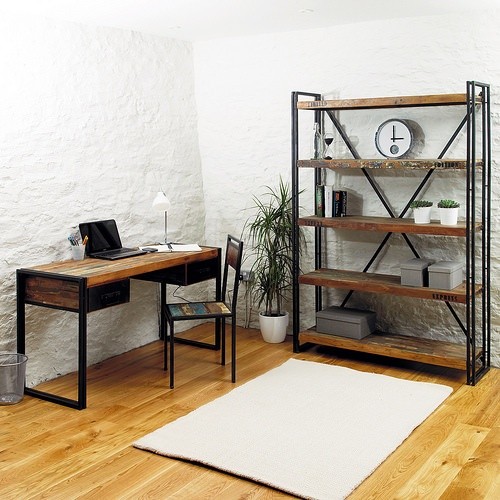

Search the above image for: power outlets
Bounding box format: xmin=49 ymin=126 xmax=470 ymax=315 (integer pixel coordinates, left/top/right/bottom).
xmin=240 ymin=271 xmax=254 ymax=281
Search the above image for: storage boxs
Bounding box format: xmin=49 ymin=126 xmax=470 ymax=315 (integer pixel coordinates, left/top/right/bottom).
xmin=315 ymin=184 xmax=348 ymax=217
xmin=316 ymin=306 xmax=377 ymax=340
xmin=400 ymin=258 xmax=464 ymax=291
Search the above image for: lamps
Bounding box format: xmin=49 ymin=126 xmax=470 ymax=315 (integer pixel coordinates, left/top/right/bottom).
xmin=152 ymin=186 xmax=171 ymax=245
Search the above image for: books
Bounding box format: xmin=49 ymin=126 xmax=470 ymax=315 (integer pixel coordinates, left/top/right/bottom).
xmin=316 ymin=184 xmax=347 ymax=218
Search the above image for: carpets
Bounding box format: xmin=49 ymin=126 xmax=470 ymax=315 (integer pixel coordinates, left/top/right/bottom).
xmin=129 ymin=358 xmax=453 ymax=500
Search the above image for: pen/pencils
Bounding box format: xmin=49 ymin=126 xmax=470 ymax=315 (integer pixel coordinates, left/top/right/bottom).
xmin=67 ymin=236 xmax=89 ymax=247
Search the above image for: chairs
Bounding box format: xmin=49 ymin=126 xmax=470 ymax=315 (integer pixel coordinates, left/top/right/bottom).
xmin=164 ymin=234 xmax=244 ymax=389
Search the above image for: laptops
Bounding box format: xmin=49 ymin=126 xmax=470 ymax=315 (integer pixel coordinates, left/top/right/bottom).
xmin=79 ymin=219 xmax=146 ymax=260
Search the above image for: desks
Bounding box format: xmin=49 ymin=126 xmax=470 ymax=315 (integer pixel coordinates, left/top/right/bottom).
xmin=16 ymin=243 xmax=222 ymax=411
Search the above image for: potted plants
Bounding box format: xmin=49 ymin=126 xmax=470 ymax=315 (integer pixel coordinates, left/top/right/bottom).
xmin=410 ymin=201 xmax=433 ymax=224
xmin=239 ymin=173 xmax=313 ymax=344
xmin=437 ymin=199 xmax=460 ymax=225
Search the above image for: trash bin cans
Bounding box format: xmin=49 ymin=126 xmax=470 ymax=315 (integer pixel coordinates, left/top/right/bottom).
xmin=0 ymin=353 xmax=28 ymax=406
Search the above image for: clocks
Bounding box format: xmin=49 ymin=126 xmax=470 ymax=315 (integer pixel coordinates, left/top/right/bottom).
xmin=374 ymin=118 xmax=413 ymax=158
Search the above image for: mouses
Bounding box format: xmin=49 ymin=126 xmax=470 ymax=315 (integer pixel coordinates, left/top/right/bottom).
xmin=142 ymin=247 xmax=158 ymax=253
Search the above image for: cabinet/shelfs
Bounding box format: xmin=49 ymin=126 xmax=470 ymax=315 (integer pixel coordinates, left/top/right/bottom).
xmin=291 ymin=81 xmax=490 ymax=387
